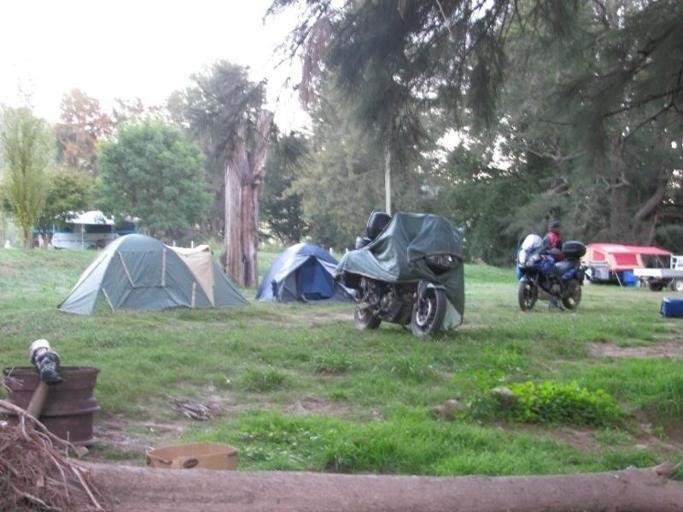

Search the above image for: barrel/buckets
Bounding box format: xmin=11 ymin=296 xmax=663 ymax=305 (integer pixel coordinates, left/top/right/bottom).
xmin=5 ymin=367 xmax=100 ymax=445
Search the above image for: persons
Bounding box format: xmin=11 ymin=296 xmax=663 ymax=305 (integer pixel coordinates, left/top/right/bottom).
xmin=543 ymin=220 xmax=565 ymax=311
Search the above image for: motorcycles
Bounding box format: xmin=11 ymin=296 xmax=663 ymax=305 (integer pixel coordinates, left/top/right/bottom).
xmin=340 ymin=208 xmax=465 ymax=341
xmin=513 ymin=230 xmax=587 ymax=311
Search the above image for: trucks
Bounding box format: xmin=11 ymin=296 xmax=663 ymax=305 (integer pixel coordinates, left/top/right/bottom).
xmin=630 ymin=252 xmax=682 ymax=292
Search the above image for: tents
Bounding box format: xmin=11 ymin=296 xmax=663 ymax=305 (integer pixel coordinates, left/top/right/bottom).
xmin=57 ymin=234 xmax=214 ymax=317
xmin=255 ymin=243 xmax=357 ymax=301
xmin=165 ymin=244 xmax=249 ymax=306
